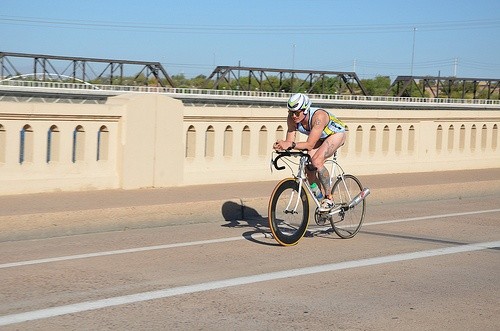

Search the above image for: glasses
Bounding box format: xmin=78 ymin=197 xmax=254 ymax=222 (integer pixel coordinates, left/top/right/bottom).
xmin=288 ymin=109 xmax=303 ymax=117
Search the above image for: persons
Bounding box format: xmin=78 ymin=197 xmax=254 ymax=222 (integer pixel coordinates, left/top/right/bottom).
xmin=273 ymin=93 xmax=349 ymax=212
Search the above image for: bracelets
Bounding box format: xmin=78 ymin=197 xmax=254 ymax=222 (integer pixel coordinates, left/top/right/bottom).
xmin=292 ymin=142 xmax=295 ymax=148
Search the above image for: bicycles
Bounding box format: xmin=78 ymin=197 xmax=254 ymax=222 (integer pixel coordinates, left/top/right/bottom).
xmin=268 ymin=141 xmax=371 ymax=247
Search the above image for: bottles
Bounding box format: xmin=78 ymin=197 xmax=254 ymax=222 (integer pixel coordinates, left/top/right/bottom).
xmin=310 ymin=182 xmax=323 ymax=201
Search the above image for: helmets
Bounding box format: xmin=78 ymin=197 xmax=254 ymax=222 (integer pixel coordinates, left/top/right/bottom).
xmin=287 ymin=92 xmax=311 ymax=115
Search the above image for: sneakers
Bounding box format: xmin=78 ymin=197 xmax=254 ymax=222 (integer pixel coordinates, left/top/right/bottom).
xmin=318 ymin=211 xmax=326 ymax=225
xmin=318 ymin=199 xmax=335 ymax=211
